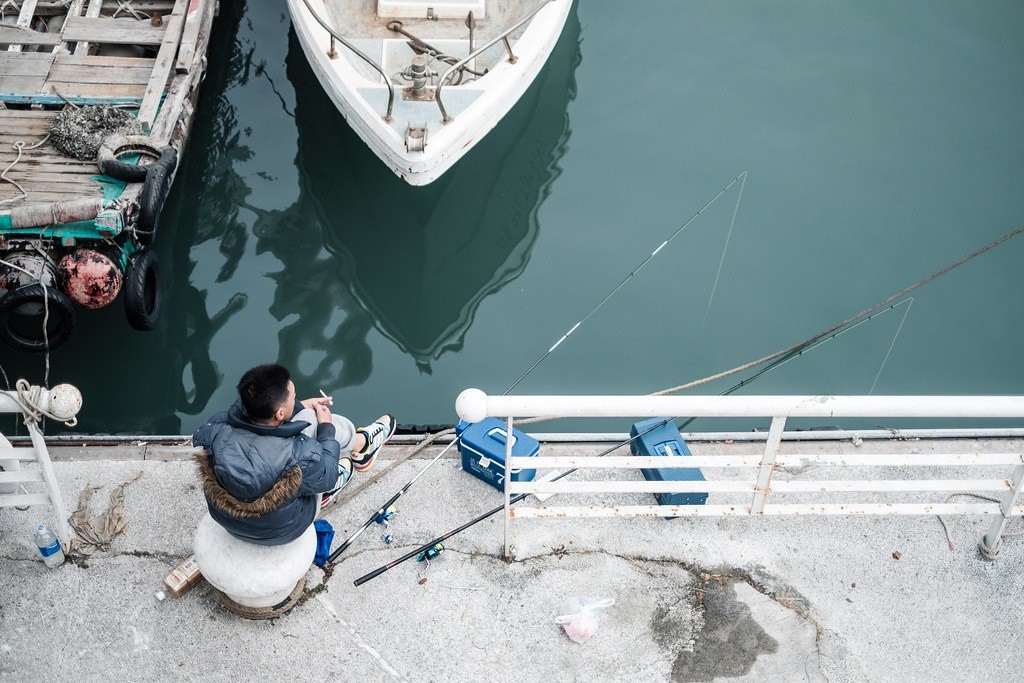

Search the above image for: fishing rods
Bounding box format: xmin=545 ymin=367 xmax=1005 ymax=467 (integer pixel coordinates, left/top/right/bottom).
xmin=326 ymin=165 xmax=749 ymax=566
xmin=352 ymin=221 xmax=1024 ymax=588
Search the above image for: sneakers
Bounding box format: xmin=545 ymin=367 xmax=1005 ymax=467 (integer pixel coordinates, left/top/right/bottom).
xmin=351 ymin=413 xmax=396 ymax=472
xmin=319 ymin=456 xmax=355 ymax=508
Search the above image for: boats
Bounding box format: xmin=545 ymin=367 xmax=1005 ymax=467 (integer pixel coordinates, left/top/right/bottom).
xmin=286 ymin=0 xmax=577 ymax=189
xmin=285 ymin=26 xmax=585 ymax=377
xmin=0 ymin=0 xmax=226 ymax=359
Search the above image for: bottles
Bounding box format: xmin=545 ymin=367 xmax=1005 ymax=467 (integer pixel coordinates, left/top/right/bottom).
xmin=33 ymin=525 xmax=65 ymax=569
xmin=155 ymin=555 xmax=203 ymax=601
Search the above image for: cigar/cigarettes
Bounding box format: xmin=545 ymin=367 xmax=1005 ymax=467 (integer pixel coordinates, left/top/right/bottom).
xmin=319 ymin=389 xmax=333 ymax=408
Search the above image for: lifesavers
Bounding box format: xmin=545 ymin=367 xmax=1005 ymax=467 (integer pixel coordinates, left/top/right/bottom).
xmin=136 ymin=164 xmax=168 ymax=245
xmin=123 ymin=250 xmax=163 ymax=331
xmin=0 ymin=284 xmax=77 ymax=352
xmin=97 ymin=133 xmax=179 ymax=183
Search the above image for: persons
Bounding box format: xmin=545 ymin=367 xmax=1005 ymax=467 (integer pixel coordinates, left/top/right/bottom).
xmin=192 ymin=365 xmax=397 ymax=545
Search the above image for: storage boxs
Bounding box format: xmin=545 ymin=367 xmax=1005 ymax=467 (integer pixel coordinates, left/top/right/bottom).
xmin=458 ymin=419 xmax=540 ymax=496
xmin=630 ymin=417 xmax=708 ymax=520
